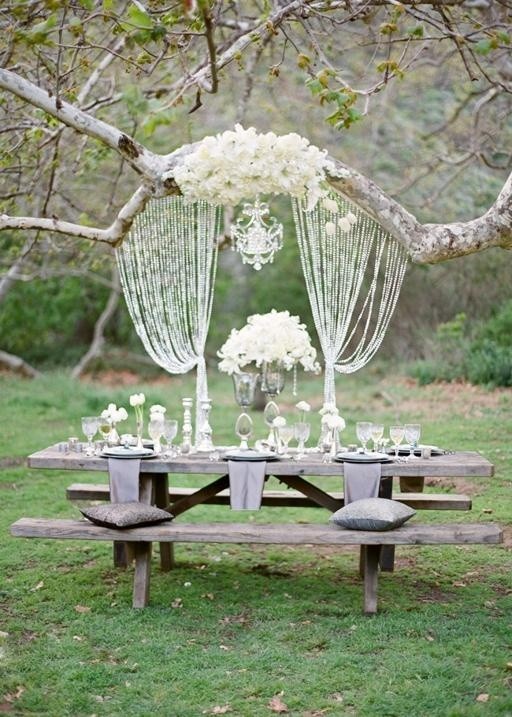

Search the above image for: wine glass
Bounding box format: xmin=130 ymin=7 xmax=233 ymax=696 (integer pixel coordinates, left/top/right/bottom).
xmin=390 ymin=422 xmax=421 ymax=461
xmin=260 ymin=359 xmax=286 ymax=443
xmin=274 ymin=421 xmax=312 ymax=459
xmin=161 ymin=419 xmax=179 ymax=453
xmin=354 ymin=419 xmax=384 ymax=452
xmin=80 ymin=413 xmax=113 ymax=454
xmin=230 ymin=372 xmax=257 ymax=450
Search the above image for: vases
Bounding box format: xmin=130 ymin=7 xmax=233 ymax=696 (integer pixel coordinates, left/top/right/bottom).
xmin=329 ymin=431 xmax=340 ymax=457
xmin=252 ymin=361 xmax=287 ymax=403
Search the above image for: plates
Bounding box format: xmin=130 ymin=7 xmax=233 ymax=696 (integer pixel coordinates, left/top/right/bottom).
xmin=223 ymin=450 xmax=275 ymax=462
xmin=334 ymin=450 xmax=390 ymax=463
xmin=98 ymin=445 xmax=160 ymax=459
xmin=119 ymin=436 xmax=154 ymax=447
xmin=392 ymin=443 xmax=441 ymax=455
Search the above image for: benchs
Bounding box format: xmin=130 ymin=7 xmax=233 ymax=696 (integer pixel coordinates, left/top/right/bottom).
xmin=9 ymin=514 xmax=506 ymax=615
xmin=65 ymin=479 xmax=474 ymax=574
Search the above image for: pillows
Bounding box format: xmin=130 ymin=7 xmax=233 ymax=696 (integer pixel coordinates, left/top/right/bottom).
xmin=327 ymin=495 xmax=419 ymax=532
xmin=77 ymin=501 xmax=177 ymax=530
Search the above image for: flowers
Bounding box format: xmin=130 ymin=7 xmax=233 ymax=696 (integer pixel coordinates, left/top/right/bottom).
xmin=318 ymin=399 xmax=339 ymax=418
xmin=100 ymin=402 xmax=130 ymax=427
xmin=216 ymin=304 xmax=322 ymax=376
xmin=146 ymin=405 xmax=166 ymax=425
xmin=324 ymin=415 xmax=345 ymax=437
xmin=130 ymin=390 xmax=147 ymax=427
xmin=271 ymin=415 xmax=287 ymax=433
xmin=294 ymin=397 xmax=310 ymax=425
xmin=171 ymin=120 xmax=359 ymax=237
